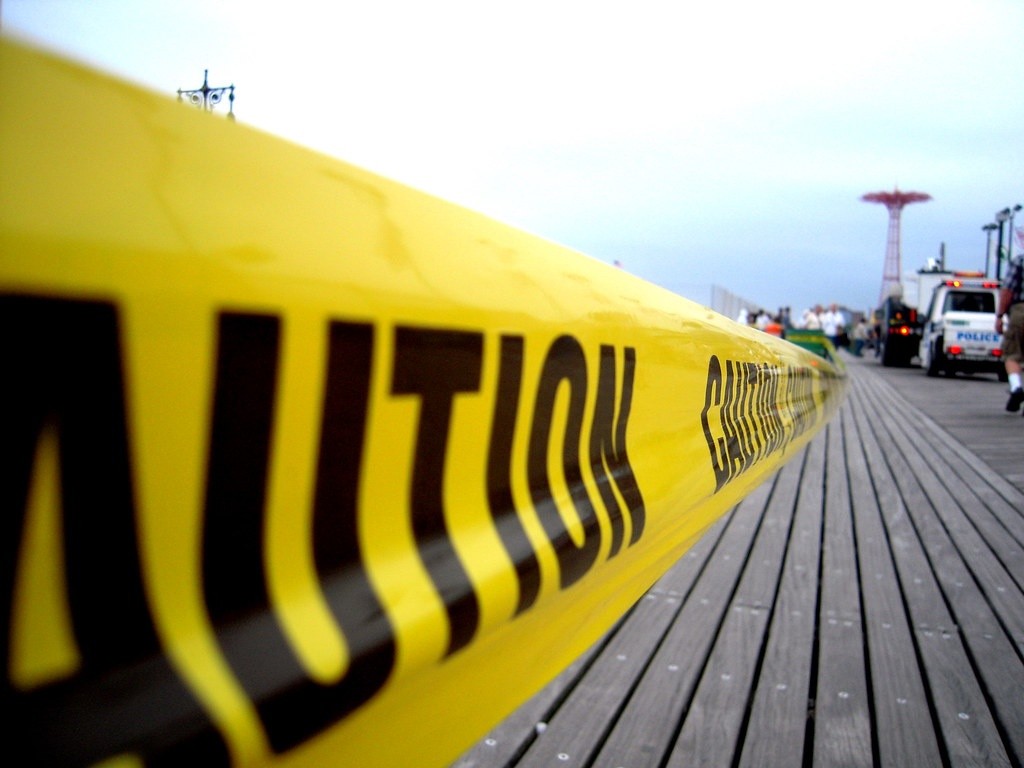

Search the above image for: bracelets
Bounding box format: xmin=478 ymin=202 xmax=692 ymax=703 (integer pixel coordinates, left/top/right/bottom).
xmin=996 ymin=313 xmax=1003 ymax=318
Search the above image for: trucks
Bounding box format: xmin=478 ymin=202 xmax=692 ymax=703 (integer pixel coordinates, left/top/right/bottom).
xmin=874 ymin=266 xmax=957 ymax=368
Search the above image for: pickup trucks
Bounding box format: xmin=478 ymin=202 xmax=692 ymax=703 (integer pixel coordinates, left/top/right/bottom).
xmin=919 ymin=280 xmax=1012 ymax=384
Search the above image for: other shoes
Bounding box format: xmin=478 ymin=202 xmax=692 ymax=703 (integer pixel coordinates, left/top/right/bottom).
xmin=1006 ymin=388 xmax=1024 ymax=411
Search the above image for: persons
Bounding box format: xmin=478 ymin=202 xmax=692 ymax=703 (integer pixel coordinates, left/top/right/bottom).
xmin=746 ymin=303 xmax=882 ymax=358
xmin=995 ymin=253 xmax=1024 ymax=417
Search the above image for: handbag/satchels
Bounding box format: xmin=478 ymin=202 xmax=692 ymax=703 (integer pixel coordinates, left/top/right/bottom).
xmin=834 ymin=333 xmax=851 ymax=348
